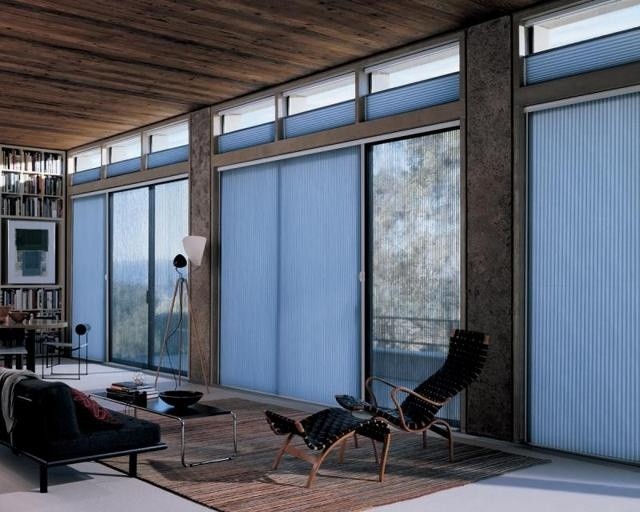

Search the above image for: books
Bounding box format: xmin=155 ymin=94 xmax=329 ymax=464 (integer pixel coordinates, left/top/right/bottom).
xmin=107 ymin=381 xmax=160 ymax=401
xmin=0 ymin=288 xmax=61 ymax=354
xmin=0 ymin=149 xmax=63 ymax=218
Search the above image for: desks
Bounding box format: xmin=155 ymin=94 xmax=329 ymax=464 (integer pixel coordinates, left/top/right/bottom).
xmin=0 ymin=322 xmax=69 ymax=373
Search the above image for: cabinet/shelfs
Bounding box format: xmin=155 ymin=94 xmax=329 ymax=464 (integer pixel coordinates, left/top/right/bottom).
xmin=0 ymin=144 xmax=66 ymax=357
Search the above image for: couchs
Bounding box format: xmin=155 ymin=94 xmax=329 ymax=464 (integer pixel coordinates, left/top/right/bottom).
xmin=0 ymin=365 xmax=169 ymax=493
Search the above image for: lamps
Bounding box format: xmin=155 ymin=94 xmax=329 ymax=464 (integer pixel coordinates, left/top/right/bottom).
xmin=155 ymin=235 xmax=210 ymax=394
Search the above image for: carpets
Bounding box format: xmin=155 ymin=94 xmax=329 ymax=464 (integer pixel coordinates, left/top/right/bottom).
xmin=82 ymin=382 xmax=551 ymax=512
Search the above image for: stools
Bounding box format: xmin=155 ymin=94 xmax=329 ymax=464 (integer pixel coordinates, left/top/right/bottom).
xmin=0 ymin=346 xmax=29 ymax=370
xmin=263 ymin=407 xmax=391 ymax=487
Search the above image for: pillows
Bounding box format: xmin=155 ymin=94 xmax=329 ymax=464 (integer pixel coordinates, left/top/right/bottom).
xmin=72 ymin=387 xmax=112 ymax=420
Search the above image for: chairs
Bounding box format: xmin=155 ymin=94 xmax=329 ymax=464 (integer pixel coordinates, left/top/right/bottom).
xmin=335 ymin=328 xmax=490 ymax=458
xmin=43 ymin=324 xmax=91 ymax=380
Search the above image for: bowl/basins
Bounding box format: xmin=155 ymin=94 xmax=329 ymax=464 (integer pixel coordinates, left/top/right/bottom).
xmin=158 ymin=391 xmax=202 ymax=407
xmin=9 ymin=311 xmax=27 ymax=322
